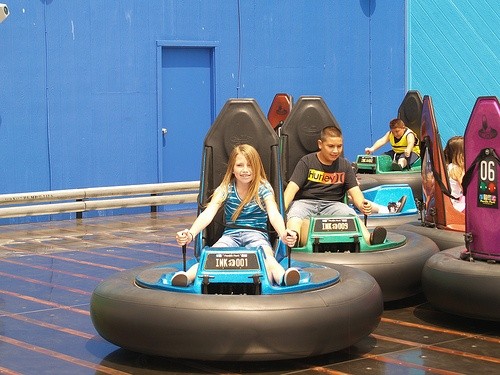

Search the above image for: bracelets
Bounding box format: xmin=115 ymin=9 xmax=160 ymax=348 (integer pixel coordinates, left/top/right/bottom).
xmin=190 ymin=232 xmax=193 ymax=243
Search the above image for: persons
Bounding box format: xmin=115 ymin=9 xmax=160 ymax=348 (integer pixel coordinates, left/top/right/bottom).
xmin=349 ymin=176 xmax=406 ymax=215
xmin=282 ymin=126 xmax=387 ymax=248
xmin=442 ymin=136 xmax=466 ymax=215
xmin=171 ymin=144 xmax=301 ymax=288
xmin=364 ymin=119 xmax=420 ymax=171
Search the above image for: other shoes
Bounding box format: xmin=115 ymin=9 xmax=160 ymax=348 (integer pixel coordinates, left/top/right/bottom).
xmin=391 ymin=162 xmax=404 ymax=171
xmin=387 ymin=195 xmax=408 ymax=214
xmin=280 ymin=267 xmax=301 ymax=286
xmin=369 ymin=225 xmax=387 ymax=245
xmin=171 ymin=270 xmax=189 ymax=287
xmin=352 ymin=162 xmax=357 ymax=171
xmin=286 ymin=231 xmax=299 ymax=249
